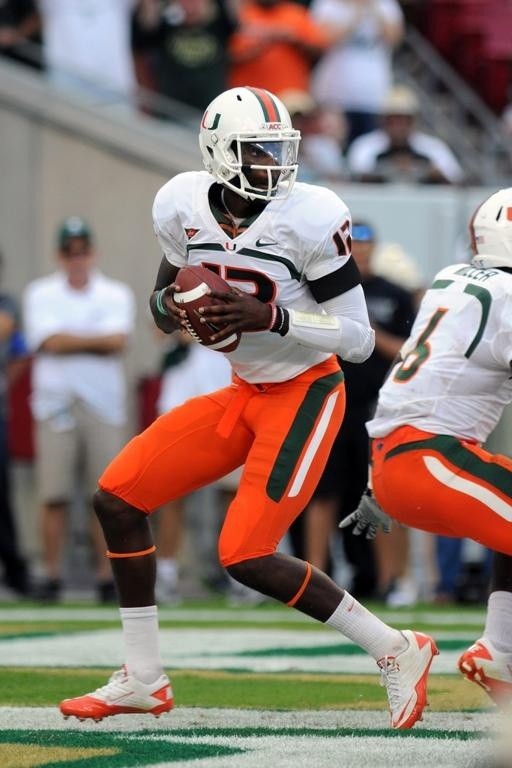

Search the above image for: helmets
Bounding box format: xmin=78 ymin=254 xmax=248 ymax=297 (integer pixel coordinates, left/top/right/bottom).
xmin=198 ymin=87 xmax=301 ymax=202
xmin=469 ymin=184 xmax=512 ymax=272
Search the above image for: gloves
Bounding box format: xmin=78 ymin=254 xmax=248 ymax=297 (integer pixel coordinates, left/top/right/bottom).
xmin=337 ymin=489 xmax=408 ymax=542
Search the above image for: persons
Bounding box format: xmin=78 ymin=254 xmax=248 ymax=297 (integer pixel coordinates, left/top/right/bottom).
xmin=340 ymin=189 xmax=512 ymax=714
xmin=60 ymin=86 xmax=440 ymax=731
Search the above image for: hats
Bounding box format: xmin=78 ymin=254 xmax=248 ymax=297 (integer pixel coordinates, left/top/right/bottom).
xmin=55 ymin=216 xmax=89 ymax=240
xmin=378 ymin=87 xmax=419 ymax=119
xmin=348 ymin=222 xmax=376 ymax=242
xmin=277 ymin=84 xmax=318 ymax=122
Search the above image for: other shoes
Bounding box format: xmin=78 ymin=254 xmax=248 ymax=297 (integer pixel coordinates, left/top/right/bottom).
xmin=153 ymin=559 xmax=182 ymax=604
xmin=38 ymin=579 xmax=66 ymax=606
xmin=228 ymin=580 xmax=270 ymax=609
xmin=455 ymin=561 xmax=488 ymax=605
xmin=98 ymin=580 xmax=119 ymax=605
xmin=386 ymin=568 xmax=443 ymax=608
xmin=349 ymin=563 xmax=380 ymax=597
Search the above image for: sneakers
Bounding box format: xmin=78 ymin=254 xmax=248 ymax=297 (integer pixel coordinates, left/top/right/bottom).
xmin=458 ymin=636 xmax=512 ymax=727
xmin=57 ymin=662 xmax=174 ymax=721
xmin=378 ymin=625 xmax=444 ymax=732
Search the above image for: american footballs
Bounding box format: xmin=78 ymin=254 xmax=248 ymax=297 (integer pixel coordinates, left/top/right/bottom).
xmin=171 ymin=264 xmax=240 ymax=352
